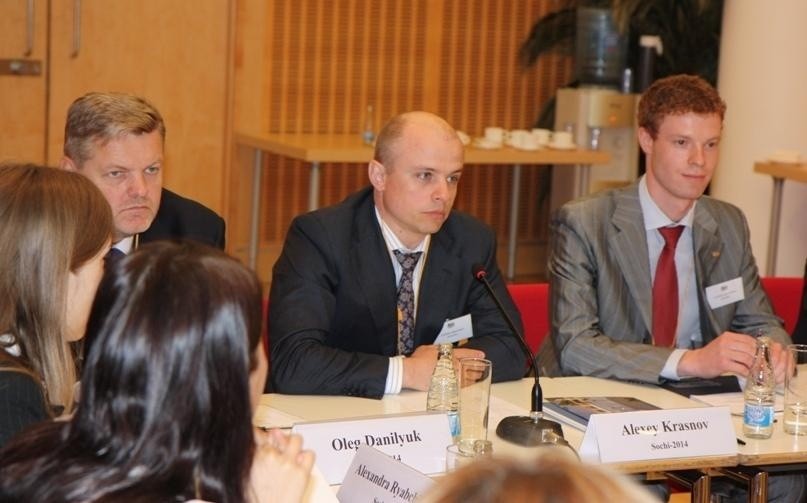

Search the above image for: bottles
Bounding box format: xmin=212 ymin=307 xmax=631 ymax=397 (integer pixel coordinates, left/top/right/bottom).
xmin=427 ymin=343 xmax=458 ymax=412
xmin=362 ymin=105 xmax=374 ymax=145
xmin=742 ymin=336 xmax=774 ymax=439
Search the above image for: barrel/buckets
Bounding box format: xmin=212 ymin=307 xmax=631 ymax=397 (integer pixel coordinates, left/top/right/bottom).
xmin=576 ymin=6 xmax=630 ymax=85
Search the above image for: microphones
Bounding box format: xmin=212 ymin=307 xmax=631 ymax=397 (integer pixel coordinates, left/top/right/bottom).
xmin=470 ymin=261 xmax=565 ymax=448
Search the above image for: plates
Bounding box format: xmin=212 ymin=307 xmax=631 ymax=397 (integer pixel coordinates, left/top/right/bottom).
xmin=478 ymin=141 xmax=578 ymax=151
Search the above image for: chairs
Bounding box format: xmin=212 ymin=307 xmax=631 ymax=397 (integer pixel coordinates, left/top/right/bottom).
xmin=506 ymin=283 xmax=550 ymax=354
xmin=761 ymin=278 xmax=804 ymax=336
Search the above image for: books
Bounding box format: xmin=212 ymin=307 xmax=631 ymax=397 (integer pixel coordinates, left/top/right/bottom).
xmin=541 ymin=397 xmax=663 ymax=432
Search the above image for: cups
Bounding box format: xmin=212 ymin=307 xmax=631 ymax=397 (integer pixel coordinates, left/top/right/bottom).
xmin=446 ymin=444 xmax=482 ymax=475
xmin=458 ymin=357 xmax=492 ymax=453
xmin=784 ymin=344 xmax=807 ymax=436
xmin=484 ymin=127 xmax=573 ymax=146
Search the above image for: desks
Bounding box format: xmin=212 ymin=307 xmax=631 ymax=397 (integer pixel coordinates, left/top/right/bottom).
xmin=753 ymin=161 xmax=807 ymax=278
xmin=252 ymin=364 xmax=807 ymax=503
xmin=237 ymin=130 xmax=613 ymax=280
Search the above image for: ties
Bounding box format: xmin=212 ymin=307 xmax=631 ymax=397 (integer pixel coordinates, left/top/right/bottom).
xmin=390 ymin=249 xmax=424 ymax=359
xmin=650 ymin=225 xmax=686 ymax=351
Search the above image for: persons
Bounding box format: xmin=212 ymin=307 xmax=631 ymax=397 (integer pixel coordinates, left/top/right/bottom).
xmin=0 ymin=242 xmax=317 ymax=503
xmin=63 ymin=89 xmax=225 ymax=378
xmin=411 ymin=445 xmax=659 ymax=502
xmin=261 ymin=110 xmax=527 ymax=399
xmin=524 ymin=74 xmax=805 ymax=503
xmin=0 ymin=163 xmax=113 ymax=441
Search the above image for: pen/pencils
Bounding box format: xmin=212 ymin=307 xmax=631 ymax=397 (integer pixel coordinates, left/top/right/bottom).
xmin=737 ymin=438 xmax=746 ymax=445
